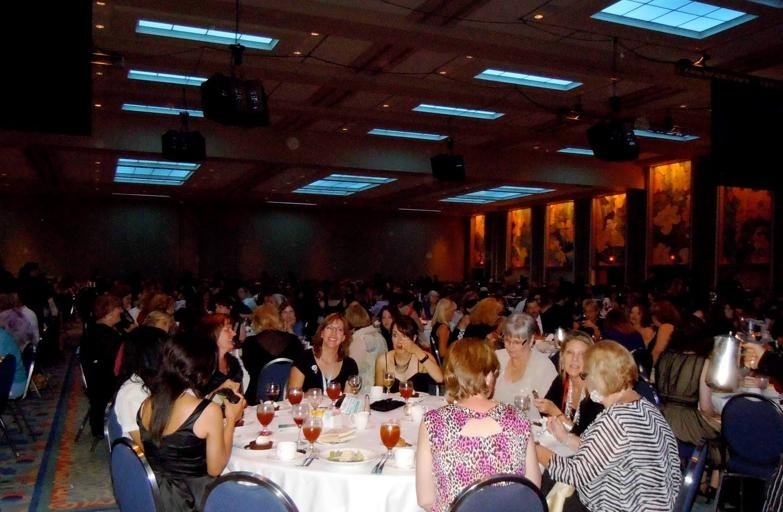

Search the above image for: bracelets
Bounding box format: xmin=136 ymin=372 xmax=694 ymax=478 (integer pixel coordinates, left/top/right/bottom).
xmin=419 ymin=352 xmax=430 ymax=363
xmin=557 ymin=412 xmax=564 ymax=420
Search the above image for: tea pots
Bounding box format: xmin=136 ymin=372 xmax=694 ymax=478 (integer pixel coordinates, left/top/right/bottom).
xmin=706 ymin=331 xmax=750 ymax=392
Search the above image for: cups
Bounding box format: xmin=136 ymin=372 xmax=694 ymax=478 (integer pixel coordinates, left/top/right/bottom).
xmin=350 ymin=412 xmax=369 ymax=431
xmin=395 ymin=449 xmax=415 ymax=468
xmin=411 ymin=405 xmax=428 ymax=423
xmin=276 ymin=441 xmax=297 ymax=461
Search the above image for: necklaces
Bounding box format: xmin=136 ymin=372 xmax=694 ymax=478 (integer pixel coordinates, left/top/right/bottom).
xmin=392 ymin=350 xmax=416 ymax=375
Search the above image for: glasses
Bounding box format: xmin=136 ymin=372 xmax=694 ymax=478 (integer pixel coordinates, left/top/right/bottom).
xmin=579 ymin=371 xmax=590 ymax=380
xmin=499 ymin=335 xmax=528 ymax=347
xmin=325 ymin=327 xmax=344 ymax=335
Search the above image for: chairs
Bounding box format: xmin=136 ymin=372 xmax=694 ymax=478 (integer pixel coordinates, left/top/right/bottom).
xmin=258 ymin=357 xmax=294 ymax=403
xmin=21 ymin=342 xmax=38 ymax=441
xmin=200 ymin=472 xmax=298 ymax=512
xmin=73 ymin=346 xmax=92 ymax=443
xmin=713 ymin=395 xmax=782 ymax=511
xmin=108 ymin=437 xmax=161 ymax=512
xmin=0 ymin=354 xmax=19 ymax=459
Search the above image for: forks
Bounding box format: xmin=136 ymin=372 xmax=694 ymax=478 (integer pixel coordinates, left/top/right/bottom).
xmin=305 ymin=454 xmax=317 ymax=466
xmin=377 ymin=457 xmax=389 ymax=474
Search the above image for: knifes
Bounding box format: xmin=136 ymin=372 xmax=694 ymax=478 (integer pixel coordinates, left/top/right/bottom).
xmin=302 ymin=454 xmax=311 ymax=466
xmin=372 ymin=455 xmax=385 ymax=472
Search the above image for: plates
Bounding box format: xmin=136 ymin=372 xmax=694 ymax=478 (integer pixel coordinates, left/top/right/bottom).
xmin=321 ymin=448 xmax=377 ymax=463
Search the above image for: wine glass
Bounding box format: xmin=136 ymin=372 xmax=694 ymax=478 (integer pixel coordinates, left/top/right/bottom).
xmin=514 ymin=395 xmax=528 ymax=412
xmin=399 ymin=382 xmax=413 ymax=406
xmin=292 ymin=404 xmax=309 ymax=445
xmin=327 ymin=382 xmax=340 ymax=407
xmin=266 ymin=383 xmax=280 ymax=405
xmin=287 ymin=387 xmax=302 ymax=405
xmin=348 ymin=375 xmax=362 ymax=394
xmin=756 ymin=378 xmax=768 ymax=393
xmin=380 ymin=423 xmax=400 ymax=456
xmin=383 ymin=372 xmax=395 ymax=395
xmin=256 ymin=404 xmax=274 ymax=436
xmin=308 ymin=388 xmax=322 ymax=410
xmin=302 ymin=417 xmax=322 ymax=450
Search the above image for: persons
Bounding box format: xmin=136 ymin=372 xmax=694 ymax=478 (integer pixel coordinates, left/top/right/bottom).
xmin=17 ymin=261 xmax=58 ymax=335
xmin=513 ymin=290 xmax=541 ymax=314
xmin=238 ymin=285 xmax=257 ymax=310
xmin=346 ymin=304 xmax=389 ymax=384
xmin=203 ymin=281 xmax=222 ymax=316
xmin=748 ymin=296 xmax=768 ymax=318
xmin=422 ymin=289 xmax=441 ymax=318
xmin=630 ymin=301 xmax=655 ymax=348
xmin=367 ymin=290 xmax=389 ymax=315
xmin=258 ymin=291 xmax=288 ymax=307
xmin=285 ymin=313 xmax=358 ymax=401
xmin=768 ymin=312 xmax=783 ymax=357
xmin=111 ymin=281 xmax=138 ymax=334
xmin=533 ymin=329 xmax=605 ymax=437
xmin=338 ymin=283 xmax=356 ymax=309
xmin=140 ymin=284 xmax=159 ymax=320
xmin=1 ymin=291 xmax=35 ymax=357
xmin=741 ymin=340 xmax=783 ymax=394
xmin=114 ymin=312 xmax=175 ymax=380
xmin=282 ymin=302 xmax=304 ymax=340
xmin=106 ymin=326 xmax=170 ymax=493
xmin=518 ymin=271 xmax=531 ymax=291
xmin=13 ymin=290 xmax=44 ymax=346
xmin=665 ymin=274 xmax=691 ymax=306
xmin=485 ymin=313 xmax=561 ymax=411
xmin=574 ymin=298 xmax=606 ymax=334
xmin=531 ymin=339 xmax=686 ymax=511
xmin=465 ymin=285 xmax=504 ymax=346
xmin=597 ymin=310 xmax=651 ymax=375
xmin=429 ymin=298 xmax=458 ymax=365
xmin=521 ymin=299 xmax=556 ymax=336
xmin=77 ymin=293 xmax=124 ymax=439
xmin=649 ymin=302 xmax=740 ymax=384
xmin=379 ymin=303 xmax=404 ymax=350
xmin=136 ymin=339 xmax=248 ymax=511
xmin=150 ymin=294 xmax=180 ymax=343
xmin=191 ymin=312 xmax=251 ymax=396
xmin=242 ymin=303 xmax=306 ymax=406
xmin=413 ymin=337 xmax=542 ymax=510
xmin=374 ymin=316 xmax=444 ymax=393
xmin=397 ymin=291 xmax=424 ymax=332
xmin=647 ymin=290 xmax=683 ymax=327
xmin=1 ymin=327 xmax=29 ymax=405
xmin=212 ymin=299 xmax=248 ymax=346
